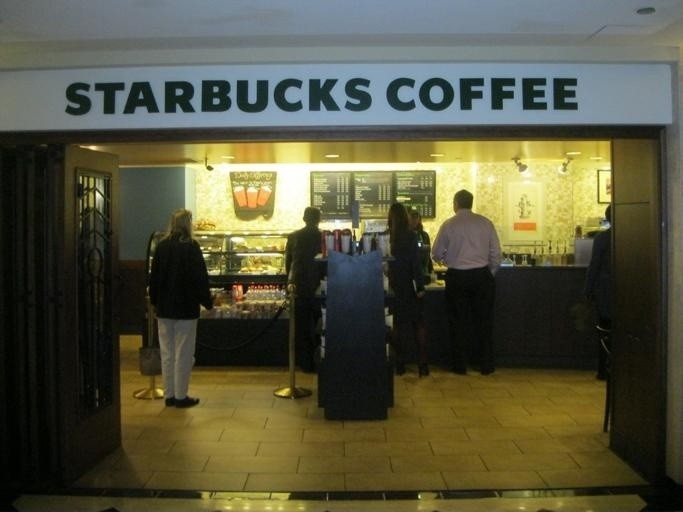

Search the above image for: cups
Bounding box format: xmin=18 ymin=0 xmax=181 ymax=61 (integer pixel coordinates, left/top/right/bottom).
xmin=234 ymin=186 xmax=272 ymax=209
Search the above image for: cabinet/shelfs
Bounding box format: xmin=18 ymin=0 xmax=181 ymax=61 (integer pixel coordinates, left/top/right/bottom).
xmin=147 ymin=231 xmax=292 ymax=366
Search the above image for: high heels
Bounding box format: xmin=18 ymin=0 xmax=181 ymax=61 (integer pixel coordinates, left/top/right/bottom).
xmin=397 ymin=361 xmax=429 ymax=378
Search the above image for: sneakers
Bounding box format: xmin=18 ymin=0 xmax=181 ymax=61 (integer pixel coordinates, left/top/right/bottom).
xmin=165 ymin=396 xmax=199 ymax=408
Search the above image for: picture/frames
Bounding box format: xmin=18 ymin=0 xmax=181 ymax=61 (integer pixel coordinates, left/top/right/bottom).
xmin=598 ymin=169 xmax=611 ymax=203
xmin=501 ymin=176 xmax=546 ymax=245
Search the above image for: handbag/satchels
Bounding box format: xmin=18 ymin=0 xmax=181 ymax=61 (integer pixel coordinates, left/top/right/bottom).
xmin=138 ymin=347 xmax=163 ymax=376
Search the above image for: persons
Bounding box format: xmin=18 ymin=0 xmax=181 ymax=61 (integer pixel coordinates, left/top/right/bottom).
xmin=150 ymin=209 xmax=215 ymax=409
xmin=284 ymin=207 xmax=326 ymax=375
xmin=380 ymin=204 xmax=430 ymax=378
xmin=433 ymin=188 xmax=501 ymax=375
xmin=583 ymin=204 xmax=614 ymax=381
xmin=408 ymin=209 xmax=433 ymax=286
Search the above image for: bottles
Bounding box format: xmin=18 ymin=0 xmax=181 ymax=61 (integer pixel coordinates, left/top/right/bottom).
xmin=232 ymin=282 xmax=286 ymax=319
xmin=531 ymin=240 xmax=568 ymax=266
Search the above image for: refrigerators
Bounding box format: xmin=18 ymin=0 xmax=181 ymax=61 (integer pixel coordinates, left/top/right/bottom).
xmin=145 ymin=229 xmax=295 ymax=276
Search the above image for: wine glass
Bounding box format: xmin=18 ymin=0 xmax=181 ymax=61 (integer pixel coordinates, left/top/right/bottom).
xmin=502 ymin=245 xmax=512 ymax=263
